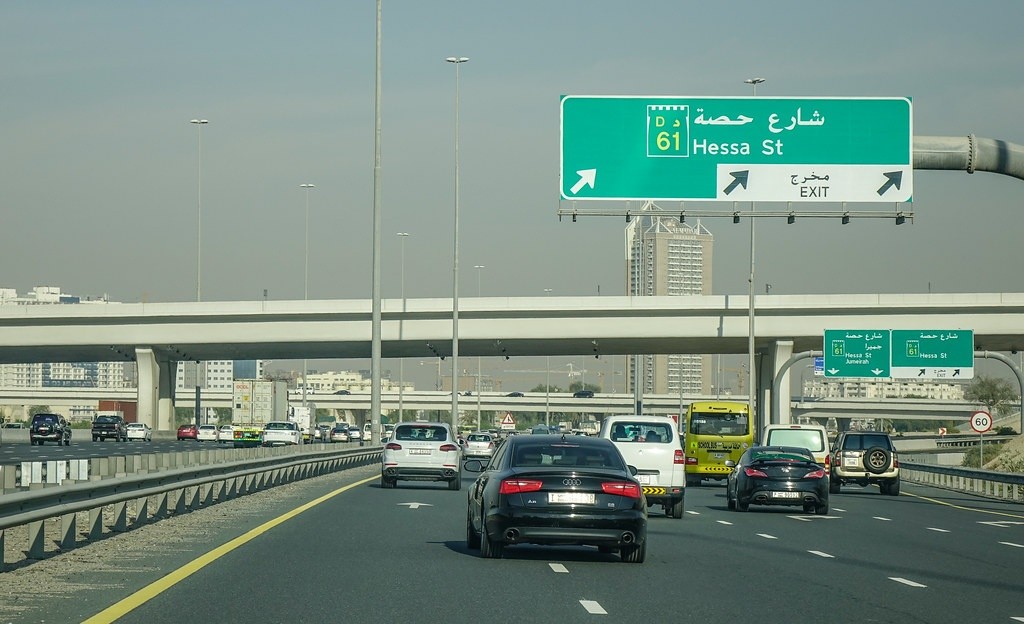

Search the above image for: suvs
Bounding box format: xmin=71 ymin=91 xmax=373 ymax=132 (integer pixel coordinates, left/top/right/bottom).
xmin=831 ymin=432 xmax=900 ymax=496
xmin=31 ymin=414 xmax=73 ymax=447
xmin=574 ymin=391 xmax=595 ymax=398
xmin=263 ymin=421 xmax=305 ymax=447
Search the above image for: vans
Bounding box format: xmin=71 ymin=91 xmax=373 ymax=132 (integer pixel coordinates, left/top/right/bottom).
xmin=363 ymin=424 xmax=386 ymax=440
xmin=762 ymin=425 xmax=831 ymax=493
xmin=383 ymin=424 xmax=394 ymax=438
xmin=600 ymin=415 xmax=685 ymax=518
xmin=295 ymin=387 xmax=316 ymax=396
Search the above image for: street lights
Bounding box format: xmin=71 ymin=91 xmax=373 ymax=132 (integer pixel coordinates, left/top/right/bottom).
xmin=474 ymin=266 xmax=487 ymax=433
xmin=746 ymin=77 xmax=767 ymax=402
xmin=397 ymin=232 xmax=409 ymax=423
xmin=446 ymin=56 xmax=469 ymax=441
xmin=192 ymin=120 xmax=208 ymax=302
xmin=300 ymin=182 xmax=316 ymax=299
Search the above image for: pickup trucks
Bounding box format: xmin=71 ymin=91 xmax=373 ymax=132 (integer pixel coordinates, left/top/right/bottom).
xmin=92 ymin=415 xmax=128 ymax=442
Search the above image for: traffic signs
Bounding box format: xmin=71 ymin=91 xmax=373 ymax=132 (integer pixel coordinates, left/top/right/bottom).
xmin=824 ymin=329 xmax=890 ymax=379
xmin=891 ymin=330 xmax=976 ymax=381
xmin=560 ymin=93 xmax=913 ymax=203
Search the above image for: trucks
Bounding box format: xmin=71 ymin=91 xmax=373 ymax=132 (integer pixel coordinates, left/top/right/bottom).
xmin=233 ymin=379 xmax=291 ymax=449
xmin=290 ymin=407 xmax=316 ymax=444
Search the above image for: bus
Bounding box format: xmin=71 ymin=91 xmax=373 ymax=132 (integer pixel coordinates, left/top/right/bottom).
xmin=684 ymin=402 xmax=754 ymax=485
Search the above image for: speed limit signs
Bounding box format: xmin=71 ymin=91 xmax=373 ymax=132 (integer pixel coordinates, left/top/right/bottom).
xmin=971 ymin=411 xmax=993 ymax=432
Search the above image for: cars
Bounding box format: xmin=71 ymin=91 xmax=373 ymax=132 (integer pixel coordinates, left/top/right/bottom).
xmin=467 ymin=435 xmax=648 ymax=564
xmin=349 ymin=428 xmax=363 ymax=441
xmin=4 ymin=423 xmax=26 ymax=430
xmin=219 ymin=425 xmax=234 ymax=444
xmin=507 ymin=392 xmax=525 ymax=397
xmin=726 ymin=446 xmax=830 ymax=514
xmin=531 ymin=422 xmax=598 ymax=438
xmin=178 ymin=425 xmax=200 ymax=441
xmin=331 ymin=429 xmax=353 ymax=443
xmin=198 ymin=425 xmax=220 ymax=442
xmin=127 ymin=423 xmax=153 ymax=442
xmin=463 ymin=433 xmax=495 ymax=460
xmin=381 ymin=423 xmax=462 ymax=491
xmin=315 ymin=427 xmax=327 ymax=442
xmin=334 ymin=390 xmax=351 ymax=396
